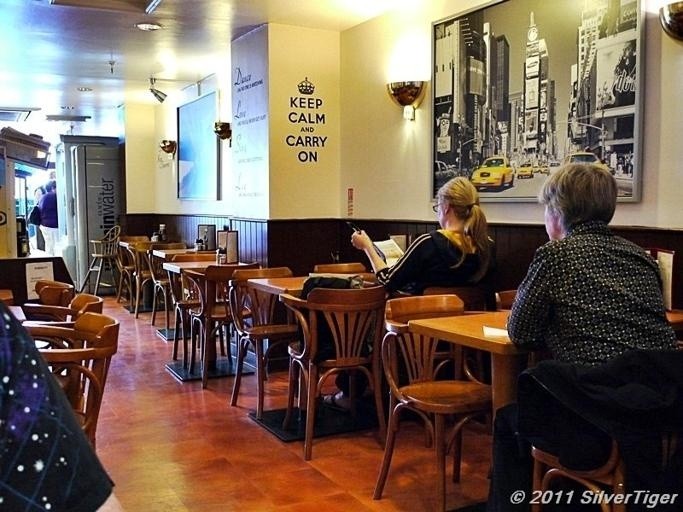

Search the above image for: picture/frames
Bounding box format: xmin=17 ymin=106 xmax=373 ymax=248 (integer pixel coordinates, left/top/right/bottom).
xmin=428 ymin=1 xmax=644 ymax=203
xmin=177 ymin=88 xmax=221 ymax=200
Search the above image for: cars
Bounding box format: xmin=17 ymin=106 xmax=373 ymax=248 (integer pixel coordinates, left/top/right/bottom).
xmin=563 ymin=153 xmax=614 ymax=175
xmin=548 ymin=162 xmax=560 ymax=175
xmin=518 ymin=164 xmax=533 ymax=179
xmin=471 ymin=156 xmax=514 ymax=191
xmin=533 ymin=165 xmax=540 ymax=172
xmin=540 ymin=165 xmax=549 ymax=174
xmin=433 ymin=160 xmax=456 ymax=182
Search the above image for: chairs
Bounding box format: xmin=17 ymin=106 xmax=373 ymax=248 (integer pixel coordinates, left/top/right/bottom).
xmin=79 ymin=225 xmax=122 ymax=299
xmin=1 ymin=276 xmax=114 ymax=455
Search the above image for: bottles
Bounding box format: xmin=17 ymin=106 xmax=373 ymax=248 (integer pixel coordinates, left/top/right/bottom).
xmin=194 ymin=239 xmax=202 ymax=251
xmin=158 ymin=224 xmax=168 ymax=241
xmin=216 ymin=249 xmax=226 ymax=264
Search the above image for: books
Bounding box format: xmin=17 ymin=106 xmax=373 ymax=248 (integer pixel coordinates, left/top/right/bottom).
xmin=347 ymin=221 xmax=404 ymax=271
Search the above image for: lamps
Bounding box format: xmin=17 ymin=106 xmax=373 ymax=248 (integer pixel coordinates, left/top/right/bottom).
xmin=149 ymin=78 xmax=167 ymax=104
xmin=159 ymin=138 xmax=176 ymax=159
xmin=386 ymin=80 xmax=427 ymax=121
xmin=212 ymin=121 xmax=231 ymax=148
xmin=658 ymin=1 xmax=683 ymax=44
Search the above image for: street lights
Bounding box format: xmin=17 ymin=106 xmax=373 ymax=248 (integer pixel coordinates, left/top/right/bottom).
xmin=456 ymin=111 xmax=465 ymax=177
xmin=596 ymin=81 xmax=608 ymax=162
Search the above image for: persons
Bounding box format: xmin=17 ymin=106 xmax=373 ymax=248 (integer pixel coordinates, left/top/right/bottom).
xmin=317 ymin=175 xmax=498 ymax=413
xmin=25 ymin=185 xmax=45 ymax=251
xmin=487 ymin=159 xmax=675 ymax=511
xmin=0 ymin=301 xmax=123 ymax=511
xmin=38 ymin=180 xmax=58 ymax=254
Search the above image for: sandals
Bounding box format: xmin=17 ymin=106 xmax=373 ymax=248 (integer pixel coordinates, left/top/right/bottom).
xmin=321 ymin=392 xmax=350 ymax=416
